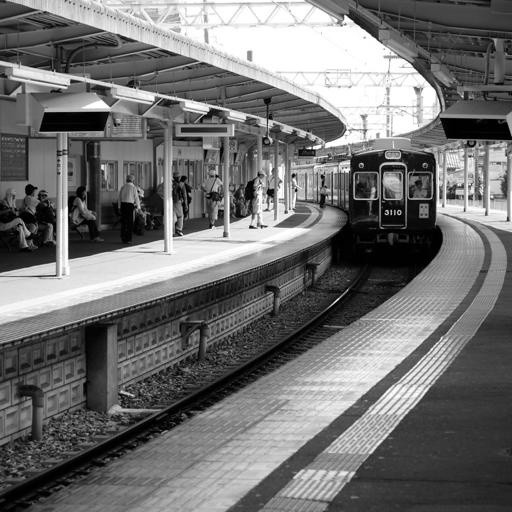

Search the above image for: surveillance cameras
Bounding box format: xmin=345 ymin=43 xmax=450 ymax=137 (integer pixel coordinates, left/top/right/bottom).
xmin=113 ymin=119 xmax=122 ymax=127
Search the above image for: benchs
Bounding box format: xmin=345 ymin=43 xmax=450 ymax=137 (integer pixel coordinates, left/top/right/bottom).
xmin=112 ymin=202 xmax=162 ymax=229
xmin=0 ymin=224 xmax=84 ymax=251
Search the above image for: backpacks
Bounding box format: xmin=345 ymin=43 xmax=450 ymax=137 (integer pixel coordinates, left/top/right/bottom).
xmin=173 ymin=182 xmax=182 ymax=203
xmin=244 ymin=177 xmax=258 ymax=200
xmin=68 ymin=196 xmax=78 ymax=213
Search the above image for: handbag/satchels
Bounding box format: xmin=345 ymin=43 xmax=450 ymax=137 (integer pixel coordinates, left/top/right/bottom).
xmin=205 ymin=191 xmax=221 ymax=201
xmin=0 ymin=205 xmax=15 ymax=224
xmin=133 ymin=213 xmax=145 ymax=236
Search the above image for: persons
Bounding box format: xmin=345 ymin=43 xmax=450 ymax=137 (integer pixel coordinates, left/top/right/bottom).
xmin=291 ymin=173 xmax=303 ymax=210
xmin=319 ymin=175 xmax=327 ymax=208
xmin=440 ymin=176 xmax=483 ymax=207
xmin=413 ymin=180 xmax=428 ymax=198
xmin=1 ymin=168 xmax=269 ymax=251
xmin=355 ymin=173 xmax=377 ymax=200
xmin=263 ymin=168 xmax=284 ymax=211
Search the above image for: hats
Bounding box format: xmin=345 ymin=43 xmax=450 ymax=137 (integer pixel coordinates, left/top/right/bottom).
xmin=126 ymin=175 xmax=135 ymax=181
xmin=40 ymin=189 xmax=48 ymax=196
xmin=173 ymin=171 xmax=182 ymax=177
xmin=25 ymin=184 xmax=37 ymax=192
xmin=258 ymin=170 xmax=267 ymax=176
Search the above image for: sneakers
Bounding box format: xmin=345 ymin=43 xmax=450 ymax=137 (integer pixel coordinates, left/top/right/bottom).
xmin=92 ymin=237 xmax=104 ymax=242
xmin=249 ymin=225 xmax=257 ymax=229
xmin=176 ymin=229 xmax=183 ymax=236
xmin=25 ymin=232 xmax=40 ymax=240
xmin=209 ymin=224 xmax=215 ymax=229
xmin=28 ymin=243 xmax=38 ymax=249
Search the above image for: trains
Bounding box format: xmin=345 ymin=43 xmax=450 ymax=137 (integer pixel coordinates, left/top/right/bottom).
xmin=293 ymin=148 xmax=438 ymax=259
